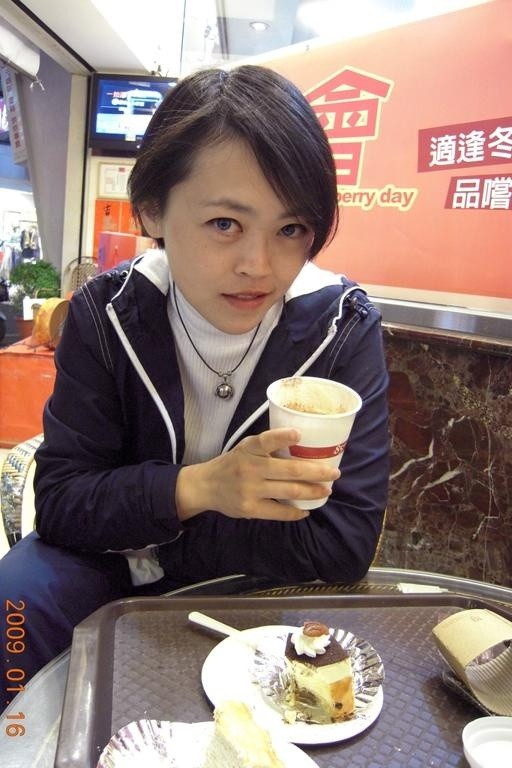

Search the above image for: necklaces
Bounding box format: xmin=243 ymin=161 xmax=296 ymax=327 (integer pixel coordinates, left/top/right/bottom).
xmin=170 ymin=277 xmax=262 ymax=400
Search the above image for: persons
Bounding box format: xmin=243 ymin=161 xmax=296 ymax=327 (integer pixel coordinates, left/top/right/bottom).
xmin=2 ymin=61 xmax=390 ymax=708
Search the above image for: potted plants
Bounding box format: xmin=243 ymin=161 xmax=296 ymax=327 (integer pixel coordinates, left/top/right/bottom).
xmin=7 ymin=259 xmax=62 ymax=322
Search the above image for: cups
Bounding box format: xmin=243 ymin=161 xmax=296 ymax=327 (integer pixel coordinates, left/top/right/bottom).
xmin=264 ymin=375 xmax=363 ymax=513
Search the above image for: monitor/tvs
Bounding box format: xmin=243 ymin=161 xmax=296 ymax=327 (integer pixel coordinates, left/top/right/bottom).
xmin=82 ymin=71 xmax=183 ymax=153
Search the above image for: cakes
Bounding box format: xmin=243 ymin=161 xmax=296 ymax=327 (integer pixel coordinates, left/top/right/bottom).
xmin=203 ymin=699 xmax=283 ymax=768
xmin=282 ymin=619 xmax=354 ymax=722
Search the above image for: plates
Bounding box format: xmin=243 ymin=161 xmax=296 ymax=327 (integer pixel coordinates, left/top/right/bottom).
xmin=199 ymin=619 xmax=384 ymax=747
xmin=188 ymin=717 xmax=322 ymax=768
